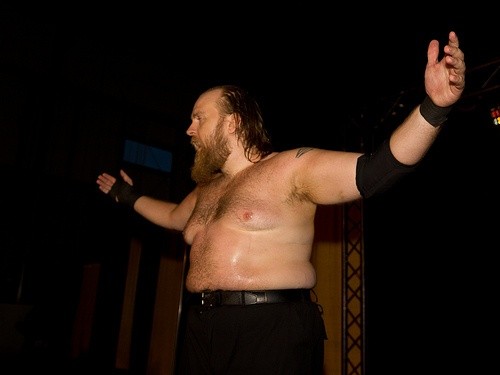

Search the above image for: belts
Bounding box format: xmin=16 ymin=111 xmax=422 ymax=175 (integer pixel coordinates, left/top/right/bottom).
xmin=181 ymin=289 xmax=311 ymax=304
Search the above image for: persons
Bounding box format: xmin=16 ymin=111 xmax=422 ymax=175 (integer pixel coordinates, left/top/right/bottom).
xmin=95 ymin=31 xmax=467 ymax=375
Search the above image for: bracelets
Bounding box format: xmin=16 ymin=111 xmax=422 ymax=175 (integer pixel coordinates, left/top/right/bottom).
xmin=421 ymin=94 xmax=452 ymax=126
